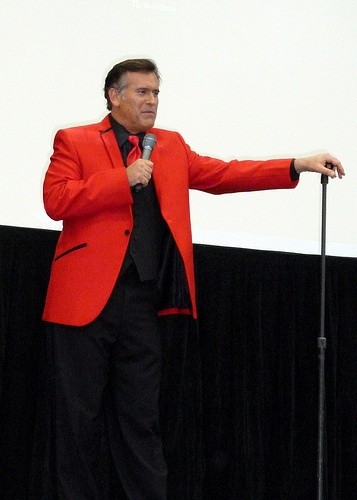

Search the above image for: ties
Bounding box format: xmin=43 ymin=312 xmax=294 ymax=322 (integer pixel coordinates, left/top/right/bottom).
xmin=126 ymin=133 xmax=143 ymax=169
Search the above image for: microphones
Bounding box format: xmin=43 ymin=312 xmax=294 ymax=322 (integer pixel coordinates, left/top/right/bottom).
xmin=133 ymin=132 xmax=157 ymax=192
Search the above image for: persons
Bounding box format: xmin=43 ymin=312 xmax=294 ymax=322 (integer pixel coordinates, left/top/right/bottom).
xmin=38 ymin=58 xmax=345 ymax=500
